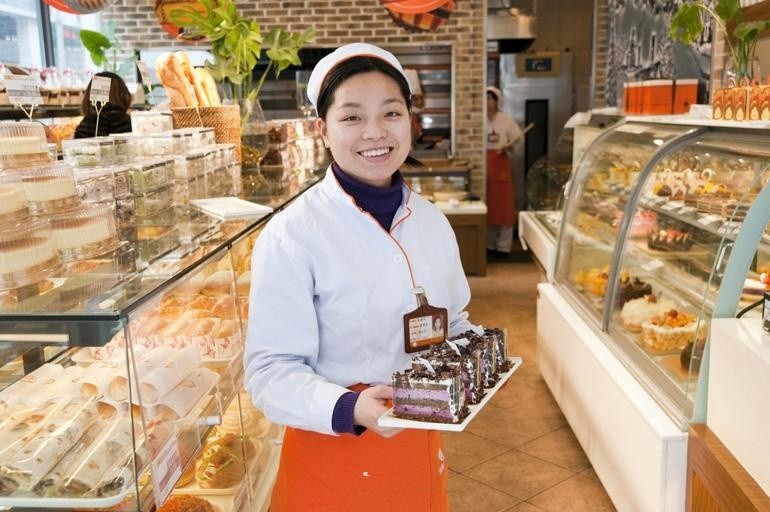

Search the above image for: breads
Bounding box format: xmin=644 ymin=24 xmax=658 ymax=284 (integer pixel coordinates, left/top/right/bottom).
xmin=140 ymin=434 xmax=255 ymax=512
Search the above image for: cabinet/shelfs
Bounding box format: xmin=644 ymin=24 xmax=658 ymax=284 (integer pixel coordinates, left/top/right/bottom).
xmin=438 ymin=213 xmax=486 ymax=277
xmin=536 ymin=113 xmax=770 ymax=512
xmin=0 ymin=103 xmax=335 ymax=512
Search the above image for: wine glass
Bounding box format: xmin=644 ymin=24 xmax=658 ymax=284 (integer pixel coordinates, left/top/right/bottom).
xmin=295 ymin=71 xmax=315 ymax=120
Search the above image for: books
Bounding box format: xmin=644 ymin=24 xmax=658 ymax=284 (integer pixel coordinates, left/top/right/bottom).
xmin=189 ymin=196 xmax=274 ymax=218
xmin=189 ymin=205 xmax=265 ymax=220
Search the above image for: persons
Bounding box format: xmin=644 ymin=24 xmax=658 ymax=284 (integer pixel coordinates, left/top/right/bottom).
xmin=486 ymin=86 xmax=526 ymax=258
xmin=241 ymin=42 xmax=472 ymax=512
xmin=74 ymin=71 xmax=132 ymax=139
xmin=402 ymin=68 xmax=445 ymax=152
xmin=432 ymin=316 xmax=445 ymax=337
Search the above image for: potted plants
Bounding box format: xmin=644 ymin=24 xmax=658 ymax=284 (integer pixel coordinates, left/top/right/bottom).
xmin=669 ymin=1 xmax=763 ymax=88
xmin=170 ymin=2 xmax=317 ymax=171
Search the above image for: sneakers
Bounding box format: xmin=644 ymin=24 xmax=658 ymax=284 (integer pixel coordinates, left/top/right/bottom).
xmin=497 ymin=252 xmax=509 ymax=258
xmin=487 ymin=248 xmax=497 ymax=257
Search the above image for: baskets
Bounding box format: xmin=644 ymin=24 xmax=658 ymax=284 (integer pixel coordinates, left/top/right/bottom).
xmin=171 ymin=104 xmax=242 ymax=166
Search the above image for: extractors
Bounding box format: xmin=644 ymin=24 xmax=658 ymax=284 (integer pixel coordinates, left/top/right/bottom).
xmin=487 ymin=0 xmax=537 ymax=53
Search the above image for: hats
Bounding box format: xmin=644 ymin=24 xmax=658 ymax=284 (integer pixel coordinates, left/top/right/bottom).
xmin=306 ymin=41 xmax=413 ymax=118
xmin=404 ymin=68 xmax=424 ymax=96
xmin=486 ymin=86 xmax=502 ymax=105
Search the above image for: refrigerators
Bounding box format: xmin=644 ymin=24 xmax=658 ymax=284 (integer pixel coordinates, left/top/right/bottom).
xmin=498 ymin=51 xmax=576 ymax=210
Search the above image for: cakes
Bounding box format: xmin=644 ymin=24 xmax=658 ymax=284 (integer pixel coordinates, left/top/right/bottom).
xmin=393 ymin=323 xmax=514 ymax=425
xmin=577 ymin=158 xmax=768 ymax=303
xmin=603 ymin=134 xmax=766 ymax=222
xmin=0 ymin=117 xmax=119 ymax=293
xmin=574 ymin=261 xmax=707 ymax=371
